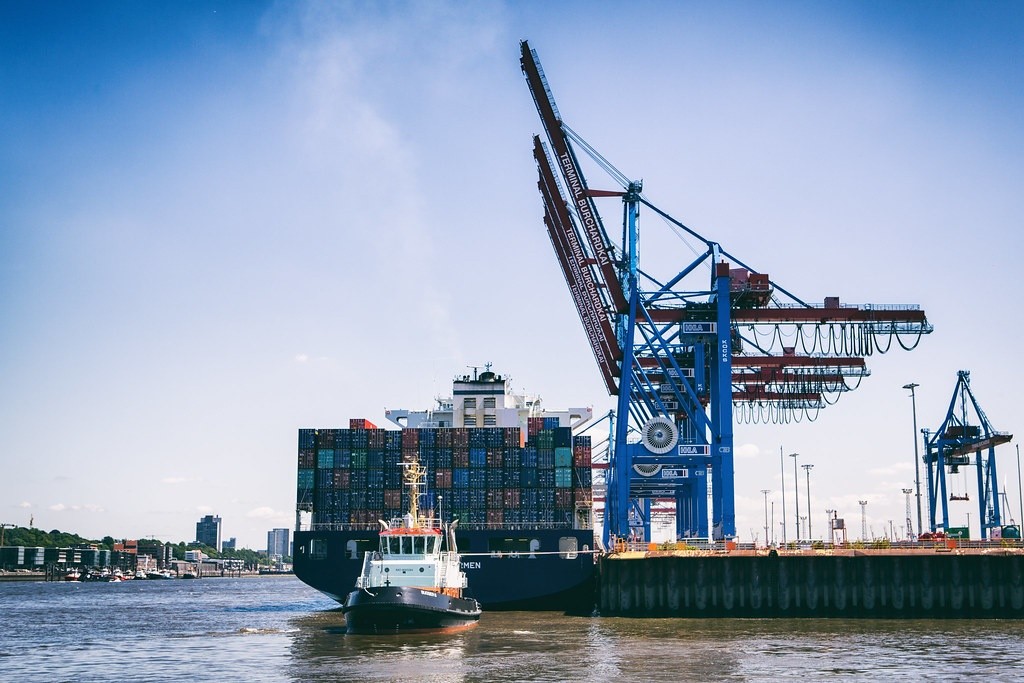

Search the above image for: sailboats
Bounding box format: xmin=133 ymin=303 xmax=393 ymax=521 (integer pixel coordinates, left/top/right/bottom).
xmin=340 ymin=449 xmax=482 ymax=637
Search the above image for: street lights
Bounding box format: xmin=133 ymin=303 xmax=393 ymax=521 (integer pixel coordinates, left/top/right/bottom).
xmin=826 ymin=509 xmax=834 ymax=539
xmin=857 ymin=499 xmax=869 ymax=542
xmin=789 ymin=453 xmax=801 ymax=540
xmin=804 ymin=462 xmax=815 ymax=542
xmin=901 ymin=487 xmax=915 ymax=541
xmin=761 ymin=489 xmax=773 ymax=549
xmin=901 ymin=382 xmax=923 ymax=540
xmin=779 ymin=446 xmax=786 ymax=542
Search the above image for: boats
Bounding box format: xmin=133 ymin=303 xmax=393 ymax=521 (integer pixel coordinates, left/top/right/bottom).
xmin=125 ymin=570 xmax=137 ymax=581
xmin=109 ymin=567 xmax=125 ymax=582
xmin=136 ymin=567 xmax=148 ymax=579
xmin=181 ymin=572 xmax=197 ymax=579
xmin=258 ymin=568 xmax=293 ymax=575
xmin=149 ymin=570 xmax=177 ymax=579
xmin=293 ymin=361 xmax=597 ymax=612
xmin=64 ymin=567 xmax=78 ymax=582
